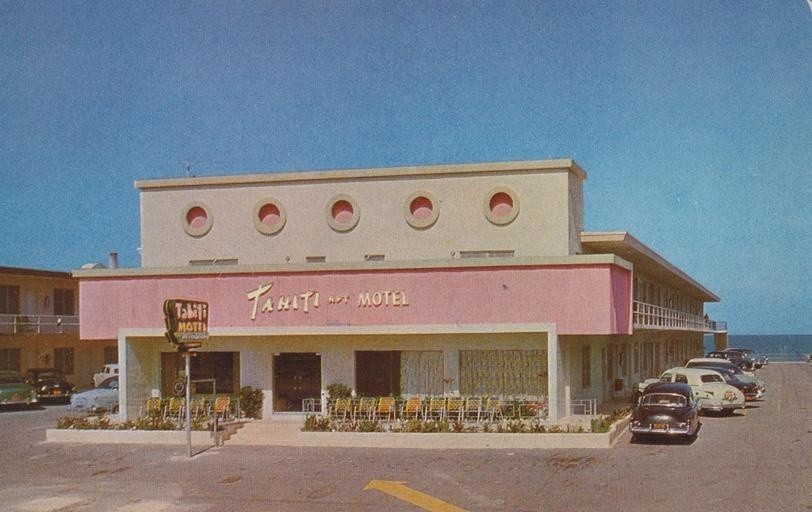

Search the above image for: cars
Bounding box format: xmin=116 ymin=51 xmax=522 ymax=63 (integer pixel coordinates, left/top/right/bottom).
xmin=629 ymin=347 xmax=768 ymax=443
xmin=0 ymin=370 xmax=38 ymax=407
xmin=66 ymin=388 xmax=118 ymax=413
xmin=23 ymin=367 xmax=78 ymax=403
xmin=91 ymin=363 xmax=119 ymax=390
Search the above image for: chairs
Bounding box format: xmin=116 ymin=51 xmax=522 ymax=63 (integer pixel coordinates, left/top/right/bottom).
xmin=331 ymin=394 xmax=503 ymax=424
xmin=141 ymin=395 xmax=229 ymax=422
